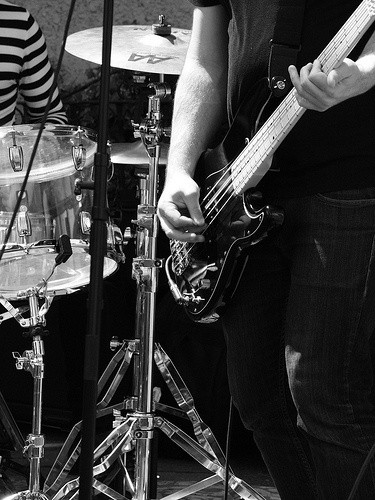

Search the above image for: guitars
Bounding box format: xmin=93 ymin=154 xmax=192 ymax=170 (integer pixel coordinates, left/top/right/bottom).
xmin=154 ymin=0 xmax=375 ymax=325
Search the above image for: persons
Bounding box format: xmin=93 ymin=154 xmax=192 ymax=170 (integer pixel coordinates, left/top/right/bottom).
xmin=0 ymin=1 xmax=68 ymax=125
xmin=156 ymin=0 xmax=375 ymax=500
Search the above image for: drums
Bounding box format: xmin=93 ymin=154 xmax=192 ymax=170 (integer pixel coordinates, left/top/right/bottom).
xmin=0 ymin=244 xmax=120 ymax=347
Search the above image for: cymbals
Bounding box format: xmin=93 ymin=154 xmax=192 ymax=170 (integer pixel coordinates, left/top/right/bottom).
xmin=63 ymin=23 xmax=192 ymax=76
xmin=107 ymin=138 xmax=170 ymax=166
